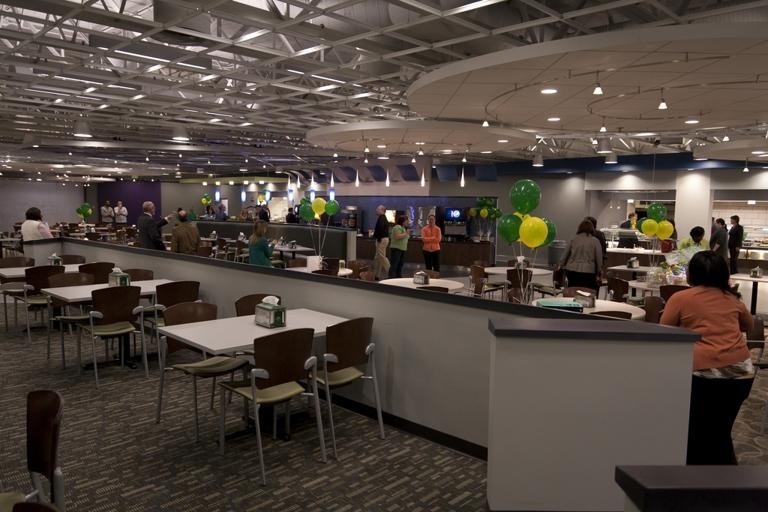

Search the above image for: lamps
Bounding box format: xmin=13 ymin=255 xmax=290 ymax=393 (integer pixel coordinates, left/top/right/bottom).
xmin=721 ymin=128 xmax=731 ymax=142
xmin=657 ymin=86 xmax=668 ymax=111
xmin=592 ymin=70 xmax=604 ymax=95
xmin=742 ymin=157 xmax=750 ymax=174
xmin=481 ymin=113 xmax=490 ymax=128
xmin=532 ymin=146 xmax=544 ymax=168
xmin=589 ymin=116 xmax=618 ymax=164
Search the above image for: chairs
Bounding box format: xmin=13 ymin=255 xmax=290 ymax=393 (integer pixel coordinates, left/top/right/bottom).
xmin=0 ymin=388 xmax=66 ymax=512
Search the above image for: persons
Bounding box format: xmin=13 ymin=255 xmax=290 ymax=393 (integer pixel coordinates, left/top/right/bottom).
xmin=727 ymin=215 xmax=744 ymax=274
xmin=136 ymin=201 xmax=176 ymax=252
xmin=239 ymin=203 xmax=270 ymax=222
xmin=553 ymin=219 xmax=604 ymax=300
xmin=615 ymin=212 xmax=640 ymax=248
xmin=217 ymin=204 xmax=227 ymax=219
xmin=710 ymin=216 xmax=718 ymax=234
xmin=585 ymin=214 xmax=607 ymax=271
xmin=170 ymin=209 xmax=202 ymax=256
xmin=247 ymin=220 xmax=278 ymax=267
xmin=286 ymin=208 xmax=296 ymax=222
xmin=709 ymin=217 xmax=728 ymax=264
xmin=322 ymin=209 xmax=336 ymax=227
xmin=658 ymin=248 xmax=756 ymax=465
xmin=114 ymin=199 xmax=129 ymax=226
xmin=420 ymin=214 xmax=441 ymax=270
xmin=679 ymin=225 xmax=709 ymax=252
xmin=20 ymin=205 xmax=53 ymax=243
xmin=387 ymin=214 xmax=414 ymax=279
xmin=205 ymin=206 xmax=216 ymax=219
xmin=100 ymin=200 xmax=117 ymax=225
xmin=464 ymin=207 xmax=476 ymax=235
xmin=372 ymin=204 xmax=390 ymax=280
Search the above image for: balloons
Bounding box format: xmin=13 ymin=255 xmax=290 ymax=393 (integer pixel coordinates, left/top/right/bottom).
xmin=299 ymin=204 xmax=315 ymax=224
xmin=647 ymin=201 xmax=667 ymax=224
xmin=75 ymin=202 xmax=92 ymax=219
xmin=498 ymin=215 xmax=523 ymax=245
xmin=510 ymin=179 xmax=543 ymax=216
xmin=311 ymin=198 xmax=327 ymax=215
xmin=469 ymin=196 xmax=503 ymax=221
xmin=325 ymin=200 xmax=339 ymax=216
xmin=642 ymin=219 xmax=657 ymax=238
xmin=655 ymin=221 xmax=674 ymax=242
xmin=300 ymin=197 xmax=305 ymax=204
xmin=258 ymin=195 xmax=264 ymax=204
xmin=512 ymin=211 xmax=531 ymax=244
xmin=636 ymin=216 xmax=646 ymax=234
xmin=201 ymin=192 xmax=212 ymax=205
xmin=537 ymin=217 xmax=558 ymax=249
xmin=519 ymin=216 xmax=548 ymax=248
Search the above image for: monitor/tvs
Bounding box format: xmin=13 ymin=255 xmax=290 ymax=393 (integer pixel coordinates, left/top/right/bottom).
xmin=444 ymin=207 xmax=466 ymax=223
